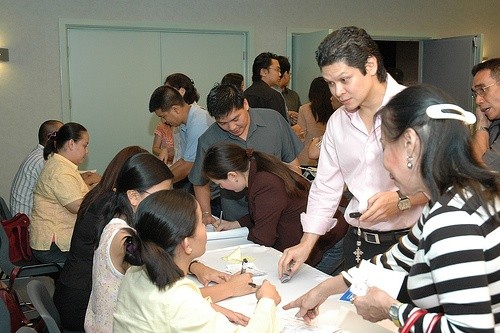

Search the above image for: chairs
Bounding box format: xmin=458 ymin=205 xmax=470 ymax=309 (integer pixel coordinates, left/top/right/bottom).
xmin=0 ymin=196 xmax=64 ymax=333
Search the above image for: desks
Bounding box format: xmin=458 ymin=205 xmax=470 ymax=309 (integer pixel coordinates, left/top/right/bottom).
xmin=184 ymin=216 xmax=401 ymax=333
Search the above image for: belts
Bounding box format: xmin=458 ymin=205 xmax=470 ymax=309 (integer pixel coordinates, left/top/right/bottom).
xmin=350 ymin=226 xmax=412 ymax=245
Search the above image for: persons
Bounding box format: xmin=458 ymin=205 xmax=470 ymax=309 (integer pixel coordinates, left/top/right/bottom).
xmin=282 ymin=83 xmax=500 ymax=333
xmin=277 ymin=24 xmax=432 ymax=281
xmin=147 ymin=53 xmax=344 ymax=226
xmin=28 ymin=122 xmax=90 ymax=262
xmin=469 ymin=57 xmax=500 ymax=175
xmin=203 ymin=142 xmax=353 ymax=279
xmin=82 ymin=152 xmax=260 ymax=333
xmin=111 ymin=188 xmax=281 ymax=333
xmin=8 ymin=119 xmax=103 ymax=216
xmin=52 ymin=145 xmax=150 ymax=333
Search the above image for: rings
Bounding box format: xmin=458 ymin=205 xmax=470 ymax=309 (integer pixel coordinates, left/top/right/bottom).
xmin=290 ymin=116 xmax=292 ymax=117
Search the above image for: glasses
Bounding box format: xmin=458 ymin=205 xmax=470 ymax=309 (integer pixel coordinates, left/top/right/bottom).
xmin=472 ymin=79 xmax=500 ymax=101
xmin=266 ymin=65 xmax=281 ymax=73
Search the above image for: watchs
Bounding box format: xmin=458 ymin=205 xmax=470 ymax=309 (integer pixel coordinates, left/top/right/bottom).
xmin=388 ymin=301 xmax=404 ymax=329
xmin=396 ymin=189 xmax=411 ymax=211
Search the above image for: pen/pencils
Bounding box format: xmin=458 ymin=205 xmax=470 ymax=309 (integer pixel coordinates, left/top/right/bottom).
xmin=240 ymin=258 xmax=248 ymax=274
xmin=220 ymin=211 xmax=223 ymax=232
xmin=248 ymin=283 xmax=260 ymax=288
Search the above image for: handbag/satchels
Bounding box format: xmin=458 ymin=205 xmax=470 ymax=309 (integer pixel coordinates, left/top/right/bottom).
xmin=2 ymin=213 xmax=36 ymax=264
xmin=0 ymin=266 xmax=28 ymax=332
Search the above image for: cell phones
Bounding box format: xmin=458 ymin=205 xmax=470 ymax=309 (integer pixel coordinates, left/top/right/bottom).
xmin=349 ymin=212 xmax=362 ymax=218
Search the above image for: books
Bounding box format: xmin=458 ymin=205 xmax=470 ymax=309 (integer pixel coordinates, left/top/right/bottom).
xmin=202 ymin=212 xmax=255 ymax=249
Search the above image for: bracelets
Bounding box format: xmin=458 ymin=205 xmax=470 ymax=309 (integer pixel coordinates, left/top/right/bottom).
xmin=202 ymin=211 xmax=211 ymax=215
xmin=473 ymin=126 xmax=490 ymax=135
xmin=312 ymin=136 xmax=319 ymax=142
xmin=187 ymin=260 xmax=197 ymax=275
